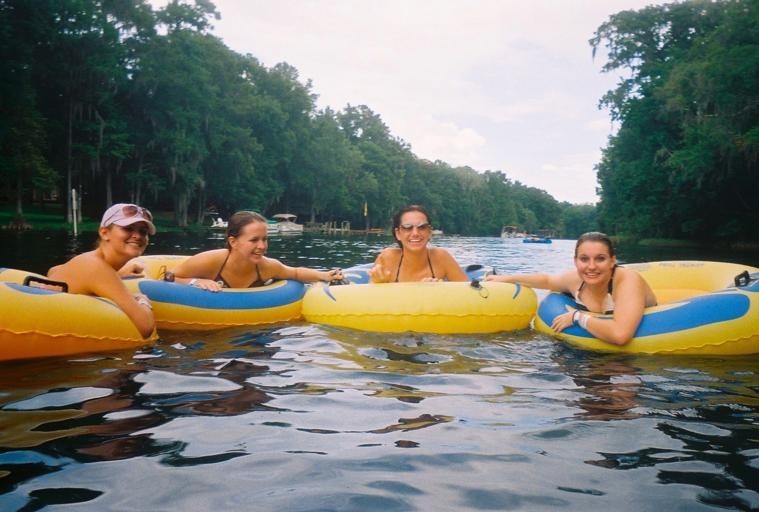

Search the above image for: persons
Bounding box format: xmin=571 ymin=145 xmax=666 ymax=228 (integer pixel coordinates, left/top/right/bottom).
xmin=164 ymin=210 xmax=344 ymax=292
xmin=365 ymin=205 xmax=469 ymax=283
xmin=486 ymin=230 xmax=657 ymax=345
xmin=41 ymin=203 xmax=158 ymax=338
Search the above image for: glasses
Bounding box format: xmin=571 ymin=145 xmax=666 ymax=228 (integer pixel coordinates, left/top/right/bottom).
xmin=398 ymin=222 xmax=428 ymax=231
xmin=103 ymin=205 xmax=152 ymax=226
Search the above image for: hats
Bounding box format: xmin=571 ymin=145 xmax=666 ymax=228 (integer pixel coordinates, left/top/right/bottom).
xmin=100 ymin=203 xmax=156 ymax=235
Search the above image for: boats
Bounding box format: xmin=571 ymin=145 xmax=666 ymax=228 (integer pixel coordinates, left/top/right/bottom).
xmin=272 ymin=213 xmax=304 ymax=232
xmin=522 ymin=236 xmax=552 ymax=244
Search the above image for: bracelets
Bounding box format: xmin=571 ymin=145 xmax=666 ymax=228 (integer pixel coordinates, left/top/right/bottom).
xmin=188 ymin=278 xmax=197 ymax=286
xmin=572 ymin=310 xmax=591 ymax=330
